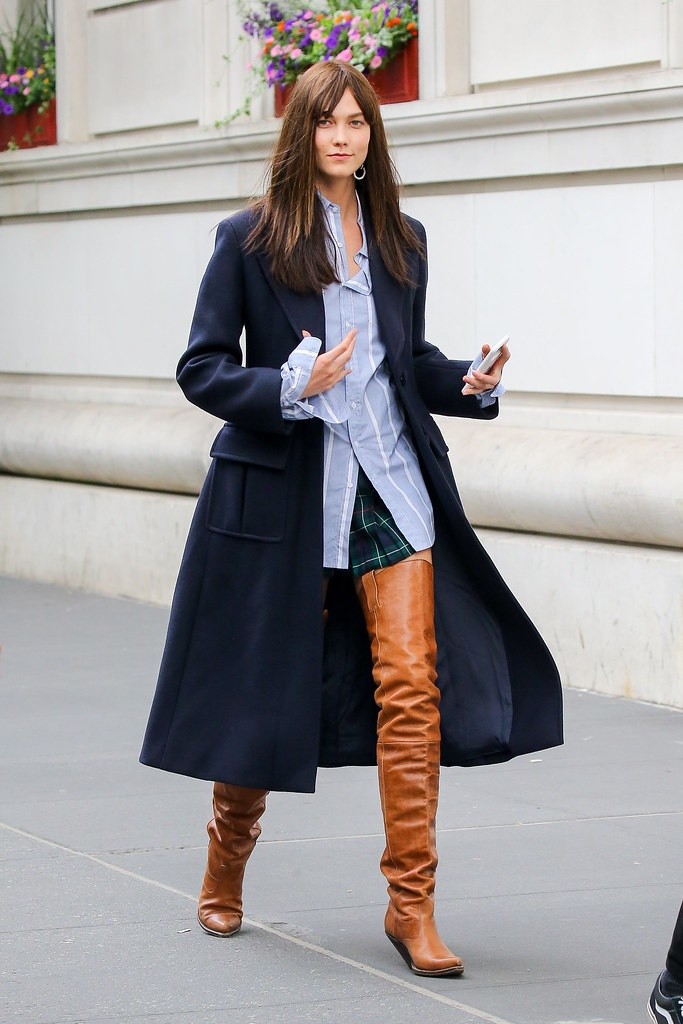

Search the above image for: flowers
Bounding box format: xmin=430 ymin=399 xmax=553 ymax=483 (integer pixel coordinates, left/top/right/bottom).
xmin=204 ymin=0 xmax=418 ymax=128
xmin=0 ymin=0 xmax=57 ymax=152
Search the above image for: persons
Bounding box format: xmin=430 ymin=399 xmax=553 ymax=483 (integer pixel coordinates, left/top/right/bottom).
xmin=140 ymin=61 xmax=564 ymax=978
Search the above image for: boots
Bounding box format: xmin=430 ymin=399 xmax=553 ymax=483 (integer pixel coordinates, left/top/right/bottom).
xmin=356 ymin=558 xmax=468 ymax=978
xmin=197 ymin=776 xmax=268 ymax=939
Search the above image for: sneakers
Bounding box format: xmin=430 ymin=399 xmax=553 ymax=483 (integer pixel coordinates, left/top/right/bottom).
xmin=648 ymin=972 xmax=683 ymax=1024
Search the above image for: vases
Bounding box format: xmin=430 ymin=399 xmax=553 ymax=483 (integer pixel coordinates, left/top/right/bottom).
xmin=273 ymin=37 xmax=419 ymax=118
xmin=0 ymin=98 xmax=56 ymax=151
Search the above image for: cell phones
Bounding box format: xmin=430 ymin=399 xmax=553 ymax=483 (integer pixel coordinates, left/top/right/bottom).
xmin=461 ymin=334 xmax=510 ymax=395
